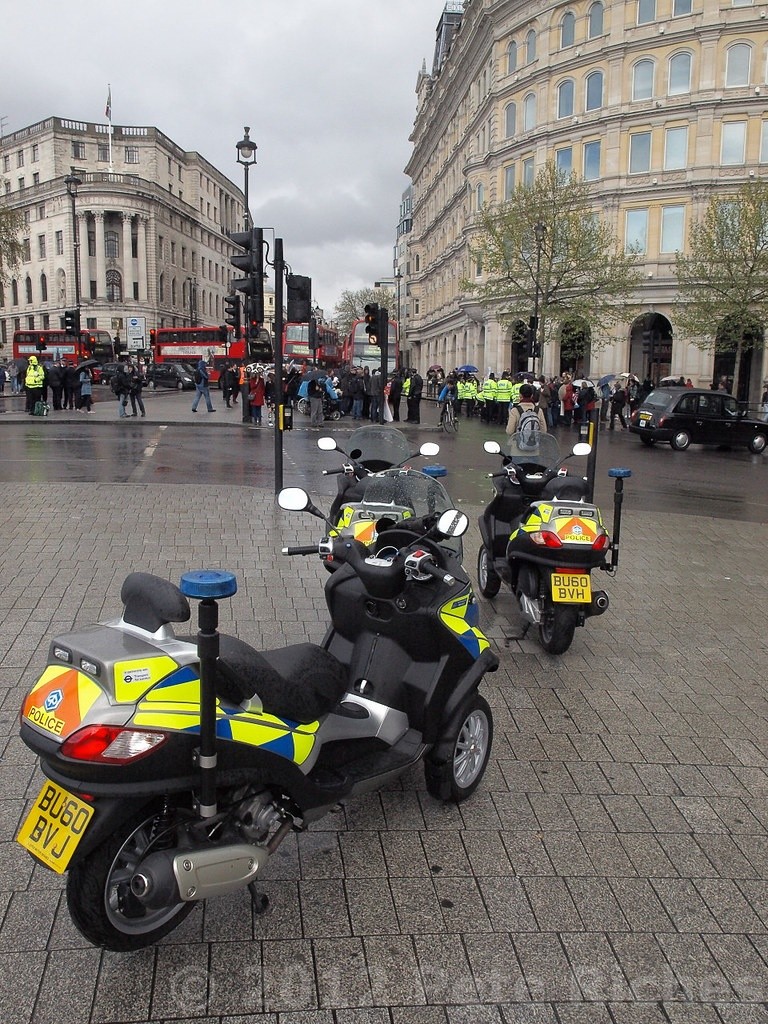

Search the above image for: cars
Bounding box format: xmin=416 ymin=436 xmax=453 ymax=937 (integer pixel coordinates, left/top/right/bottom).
xmin=101 ymin=363 xmax=145 ymax=386
xmin=627 ymin=387 xmax=768 ymax=454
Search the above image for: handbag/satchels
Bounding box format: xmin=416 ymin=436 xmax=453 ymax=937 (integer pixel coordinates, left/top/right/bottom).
xmin=192 ymin=370 xmax=201 ymax=384
xmin=248 ymin=393 xmax=256 ymax=400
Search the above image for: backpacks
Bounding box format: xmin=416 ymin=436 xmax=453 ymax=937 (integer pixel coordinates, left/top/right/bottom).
xmin=515 ymin=405 xmax=541 ymax=452
xmin=34 ymin=401 xmax=47 ymax=416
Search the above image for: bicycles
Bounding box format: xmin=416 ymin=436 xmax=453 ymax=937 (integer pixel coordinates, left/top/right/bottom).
xmin=435 ymin=398 xmax=460 ymax=435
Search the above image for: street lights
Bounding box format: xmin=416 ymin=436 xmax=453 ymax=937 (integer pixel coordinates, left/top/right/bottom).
xmin=235 ymin=126 xmax=258 ymax=232
xmin=62 ymin=172 xmax=85 ymax=331
xmin=532 ymin=220 xmax=547 ymax=374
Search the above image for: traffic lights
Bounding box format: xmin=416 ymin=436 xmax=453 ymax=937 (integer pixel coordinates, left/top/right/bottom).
xmin=64 ymin=309 xmax=79 ymax=337
xmin=642 ymin=328 xmax=655 ymax=356
xmin=248 ymin=321 xmax=262 ymax=338
xmin=229 ymin=225 xmax=265 ymax=321
xmin=523 ymin=329 xmax=534 ymax=353
xmin=224 ymin=294 xmax=242 ymax=338
xmin=36 ymin=334 xmax=47 ymax=350
xmin=149 ymin=329 xmax=155 ymax=344
xmin=364 ymin=300 xmax=379 ymax=334
xmin=84 ymin=335 xmax=96 ymax=350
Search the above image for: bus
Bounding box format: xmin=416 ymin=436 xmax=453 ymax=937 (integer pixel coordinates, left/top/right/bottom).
xmin=282 ymin=322 xmax=343 ymax=370
xmin=351 ymin=319 xmax=401 ymax=375
xmin=155 ymin=326 xmax=273 ymax=385
xmin=338 ymin=333 xmax=351 ymax=366
xmin=12 ymin=328 xmax=114 ymax=375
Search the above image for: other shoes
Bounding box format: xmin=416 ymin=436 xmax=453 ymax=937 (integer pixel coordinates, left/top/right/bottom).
xmin=209 ymin=410 xmax=216 ymax=412
xmin=88 ymin=410 xmax=95 ymax=414
xmin=131 ymin=413 xmax=137 ymax=416
xmin=192 ymin=409 xmax=197 ymax=412
xmin=76 ymin=409 xmax=85 ymax=413
xmin=141 ymin=411 xmax=145 ymax=417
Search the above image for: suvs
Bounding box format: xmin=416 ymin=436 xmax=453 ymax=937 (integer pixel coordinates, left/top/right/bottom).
xmin=146 ymin=362 xmax=197 ymax=391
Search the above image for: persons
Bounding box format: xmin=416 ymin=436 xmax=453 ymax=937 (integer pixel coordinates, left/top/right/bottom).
xmin=426 ymin=368 xmax=768 ymax=432
xmin=0 ymin=355 xmax=146 ymax=418
xmin=505 ymin=384 xmax=547 ymax=464
xmin=192 ymin=361 xmax=424 ymax=428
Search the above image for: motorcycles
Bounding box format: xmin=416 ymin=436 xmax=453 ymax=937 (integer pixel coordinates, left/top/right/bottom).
xmin=476 ymin=430 xmax=633 ymax=655
xmin=15 ymin=463 xmax=506 ymax=955
xmin=316 ymin=423 xmax=450 ymax=578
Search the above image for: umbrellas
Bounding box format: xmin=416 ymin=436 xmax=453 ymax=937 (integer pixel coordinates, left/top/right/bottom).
xmin=430 ymin=365 xmax=681 ymax=388
xmin=75 ymin=359 xmax=100 ymax=372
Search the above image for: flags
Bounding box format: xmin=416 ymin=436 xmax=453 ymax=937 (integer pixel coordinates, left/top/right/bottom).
xmin=105 ymin=85 xmax=112 ymax=121
